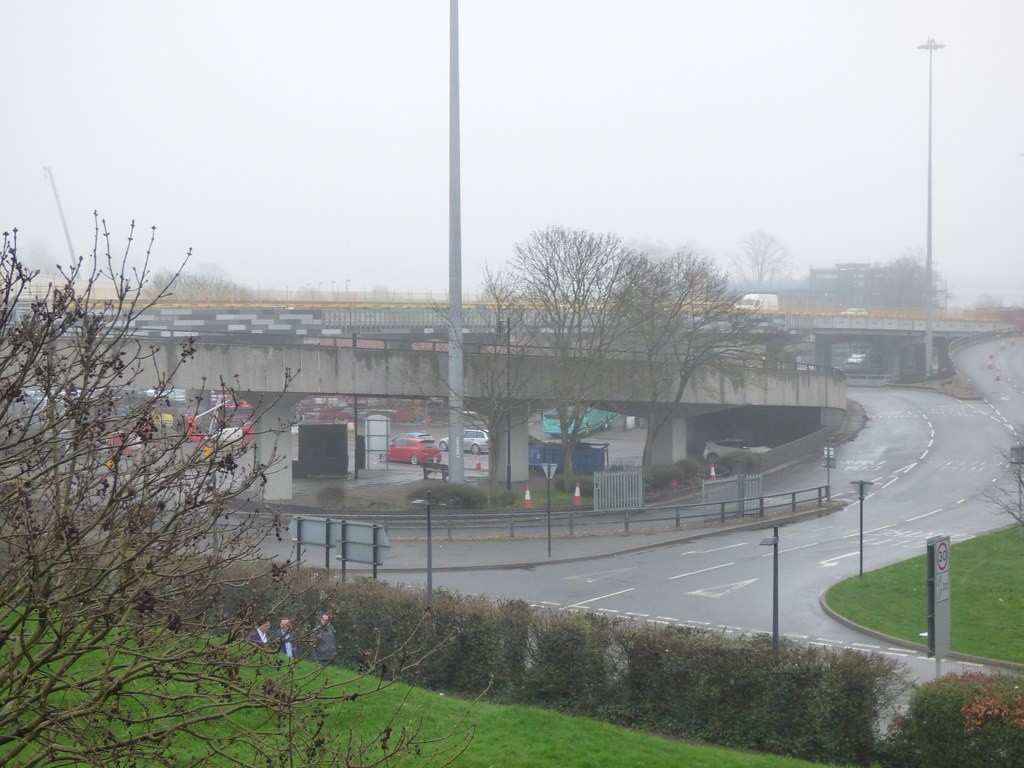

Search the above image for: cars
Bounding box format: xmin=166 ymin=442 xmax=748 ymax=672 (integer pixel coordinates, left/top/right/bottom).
xmin=379 ymin=437 xmax=442 ymax=466
xmin=838 ymin=308 xmax=868 ymax=317
xmin=145 ymin=387 xmax=255 ymax=444
xmin=392 ymin=432 xmax=432 ymax=440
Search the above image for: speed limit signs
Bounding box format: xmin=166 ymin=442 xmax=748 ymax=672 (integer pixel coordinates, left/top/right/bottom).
xmin=936 ymin=542 xmax=949 ymax=570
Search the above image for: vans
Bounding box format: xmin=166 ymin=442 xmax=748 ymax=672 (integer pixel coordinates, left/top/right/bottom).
xmin=736 ymin=293 xmax=781 ymax=313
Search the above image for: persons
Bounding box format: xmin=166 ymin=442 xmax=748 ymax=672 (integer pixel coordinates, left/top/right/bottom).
xmin=312 ymin=614 xmax=338 ymax=665
xmin=249 ymin=616 xmax=300 ymax=659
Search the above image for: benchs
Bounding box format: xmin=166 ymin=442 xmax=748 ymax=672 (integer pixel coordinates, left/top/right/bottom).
xmin=420 ymin=461 xmax=448 ymax=483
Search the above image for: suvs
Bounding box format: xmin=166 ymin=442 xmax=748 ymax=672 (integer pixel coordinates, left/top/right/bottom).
xmin=703 ymin=439 xmax=771 ymax=465
xmin=439 ymin=429 xmax=491 ymax=454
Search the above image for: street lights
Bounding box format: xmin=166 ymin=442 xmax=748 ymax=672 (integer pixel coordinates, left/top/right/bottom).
xmin=917 ymin=36 xmax=945 ymax=377
xmin=848 ymin=481 xmax=874 ymax=576
xmin=757 ymin=524 xmax=780 ymax=659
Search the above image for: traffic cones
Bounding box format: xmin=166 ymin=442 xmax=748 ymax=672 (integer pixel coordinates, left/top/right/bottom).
xmin=709 ymin=462 xmax=717 ymax=481
xmin=523 ymin=483 xmax=533 ymax=510
xmin=570 ymin=482 xmax=584 ymax=506
xmin=476 ymin=453 xmax=481 ymax=470
